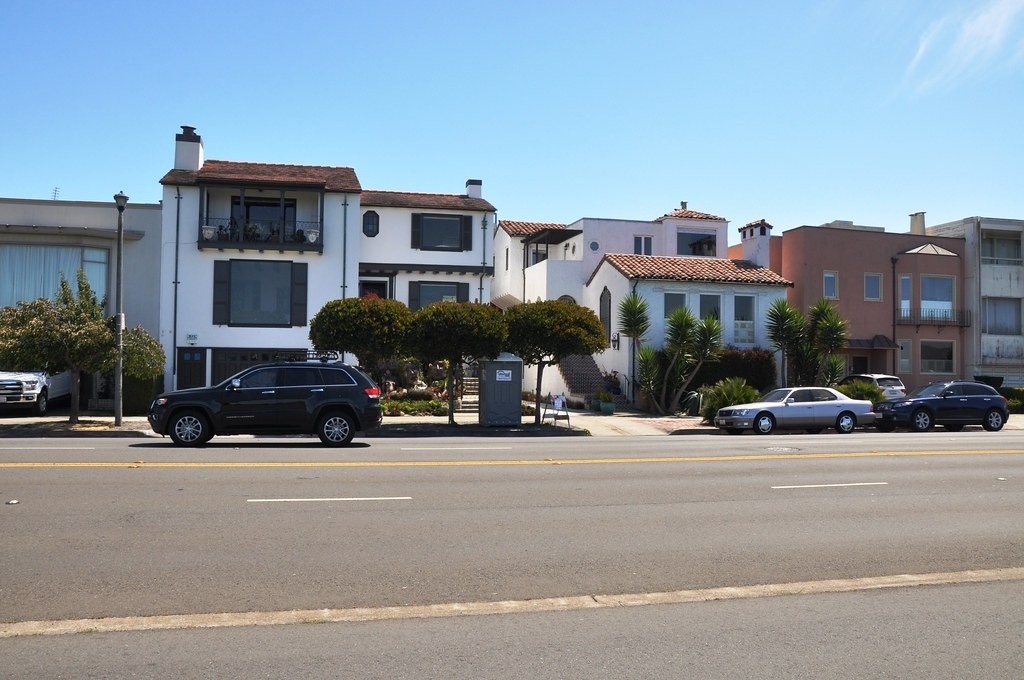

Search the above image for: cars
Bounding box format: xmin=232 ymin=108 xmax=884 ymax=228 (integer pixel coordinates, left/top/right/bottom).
xmin=714 ymin=386 xmax=876 ymax=435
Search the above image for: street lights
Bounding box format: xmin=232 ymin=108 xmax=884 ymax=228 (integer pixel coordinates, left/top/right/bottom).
xmin=890 ymin=255 xmax=899 ymax=376
xmin=114 ymin=190 xmax=130 ymax=427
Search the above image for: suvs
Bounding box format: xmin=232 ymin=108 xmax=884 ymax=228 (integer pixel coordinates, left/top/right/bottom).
xmin=873 ymin=379 xmax=1010 ymax=433
xmin=0 ymin=356 xmax=73 ymax=417
xmin=146 ymin=349 xmax=384 ymax=449
xmin=831 ymin=372 xmax=908 ymax=401
